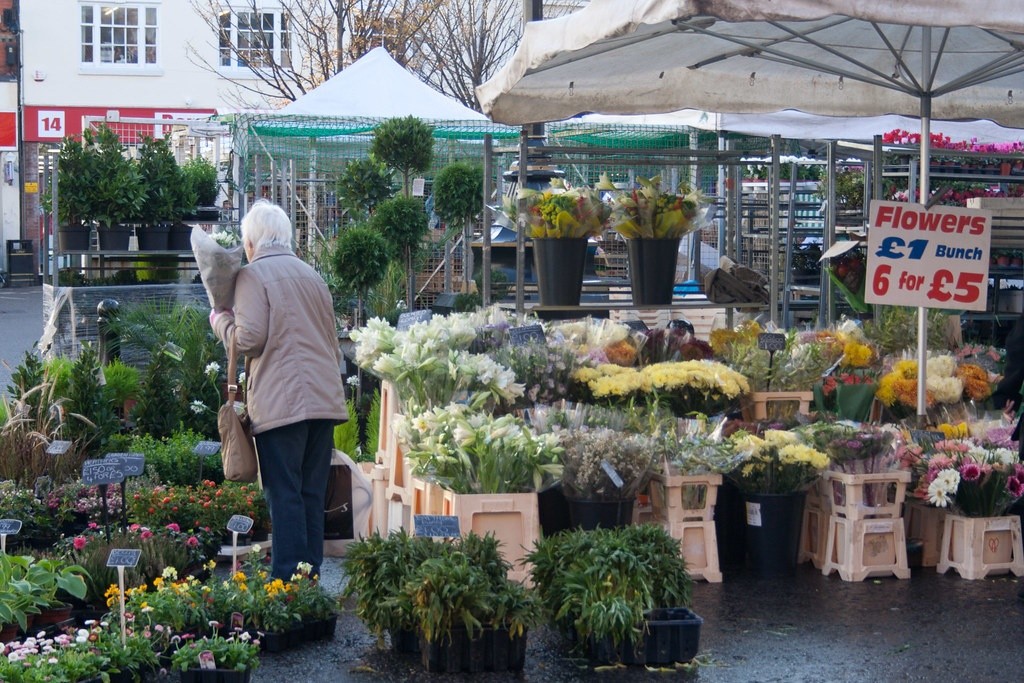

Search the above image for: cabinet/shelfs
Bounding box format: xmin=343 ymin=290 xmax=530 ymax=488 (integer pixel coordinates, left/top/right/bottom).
xmin=484 ymin=133 xmax=781 ymax=330
xmin=781 ymin=164 xmax=824 ymax=330
xmin=742 ymin=193 xmax=824 ymax=260
xmin=824 ymin=142 xmax=871 ymax=332
xmin=874 ymin=134 xmax=1023 ymax=344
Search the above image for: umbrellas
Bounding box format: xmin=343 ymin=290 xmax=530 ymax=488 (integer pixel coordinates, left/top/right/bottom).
xmin=474 ymin=0 xmax=1024 ymax=424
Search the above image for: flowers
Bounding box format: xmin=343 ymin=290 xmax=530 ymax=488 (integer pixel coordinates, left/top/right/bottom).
xmin=349 ymin=127 xmax=1024 ymax=520
xmin=0 ymin=468 xmax=336 ymax=683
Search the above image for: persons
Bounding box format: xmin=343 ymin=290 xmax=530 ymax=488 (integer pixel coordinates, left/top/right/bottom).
xmin=209 ymin=199 xmax=353 ymax=587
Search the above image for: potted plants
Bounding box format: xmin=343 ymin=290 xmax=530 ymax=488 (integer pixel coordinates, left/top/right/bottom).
xmin=41 ymin=125 xmax=223 ymax=252
xmin=338 ymin=524 xmax=702 ymax=672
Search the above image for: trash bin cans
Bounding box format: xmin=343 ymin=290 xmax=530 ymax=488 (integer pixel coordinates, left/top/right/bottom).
xmin=5 ymin=238 xmax=35 ymax=286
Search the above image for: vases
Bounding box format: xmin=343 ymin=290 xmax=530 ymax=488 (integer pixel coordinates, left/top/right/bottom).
xmin=5 ymin=541 xmax=18 ymax=553
xmin=627 ymin=237 xmax=681 ymax=307
xmin=225 ymin=531 xmax=252 ymax=547
xmin=31 ymin=535 xmax=61 ymax=551
xmin=531 ymin=238 xmax=589 ymax=309
xmin=182 ymin=667 xmax=252 ymax=682
xmin=26 ymin=613 xmax=37 ymax=628
xmin=152 ymin=621 xmax=216 ymax=665
xmin=252 ymin=526 xmax=271 ymax=542
xmin=90 ymin=661 xmax=139 ymax=682
xmin=0 ymin=621 xmax=21 ymax=644
xmin=367 ymin=378 xmax=1024 ymax=588
xmin=37 ymin=603 xmax=75 ymax=625
xmin=967 ymin=197 xmax=1024 ymax=251
xmin=225 ymin=613 xmax=334 ymax=652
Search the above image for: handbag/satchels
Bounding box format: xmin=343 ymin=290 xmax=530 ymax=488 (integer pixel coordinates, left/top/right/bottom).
xmin=323 ymin=448 xmax=373 ymax=559
xmin=217 ymin=325 xmax=258 ymax=483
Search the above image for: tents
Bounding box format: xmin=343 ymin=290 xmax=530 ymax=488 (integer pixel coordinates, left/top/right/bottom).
xmin=539 ymin=108 xmax=1024 ymax=280
xmin=168 ymin=47 xmax=522 ymax=299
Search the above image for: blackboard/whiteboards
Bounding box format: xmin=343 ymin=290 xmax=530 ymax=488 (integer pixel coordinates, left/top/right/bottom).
xmin=0 ymin=518 xmax=23 ymax=535
xmin=413 ymin=515 xmax=461 ymax=536
xmin=616 ymin=320 xmax=649 ymax=330
xmin=104 ymin=454 xmax=145 ymax=476
xmin=46 ymin=440 xmax=72 ymax=455
xmin=757 ymin=333 xmax=787 ymax=351
xmin=195 ymin=441 xmax=221 ymax=456
xmin=82 ymin=458 xmax=126 ymax=484
xmin=106 ymin=549 xmax=142 ymax=568
xmin=508 ymin=324 xmax=547 ymax=346
xmin=396 ymin=309 xmax=432 ymax=333
xmin=226 ymin=515 xmax=254 ymax=533
